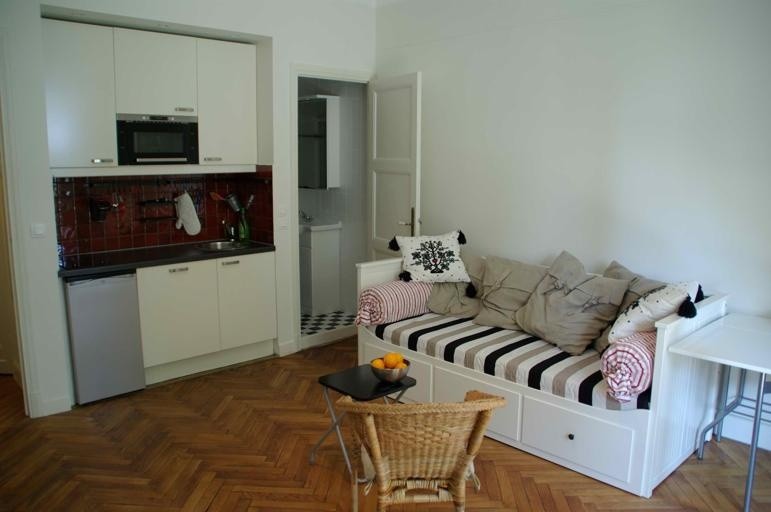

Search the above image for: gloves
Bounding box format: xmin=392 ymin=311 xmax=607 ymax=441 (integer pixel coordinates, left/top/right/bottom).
xmin=174 ymin=193 xmax=202 ymax=238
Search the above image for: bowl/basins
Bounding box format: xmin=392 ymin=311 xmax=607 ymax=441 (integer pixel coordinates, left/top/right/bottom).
xmin=372 ymin=357 xmax=411 ymax=383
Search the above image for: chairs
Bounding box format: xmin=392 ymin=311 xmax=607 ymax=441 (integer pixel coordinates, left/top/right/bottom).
xmin=335 ymin=389 xmax=507 ymax=511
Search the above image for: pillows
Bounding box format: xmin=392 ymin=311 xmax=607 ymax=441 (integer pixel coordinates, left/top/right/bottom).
xmin=607 ymin=278 xmax=705 ymax=343
xmin=513 ymin=248 xmax=629 ymax=356
xmin=592 ymin=260 xmax=671 ymax=356
xmin=600 ymin=327 xmax=659 ymax=404
xmin=425 ymin=249 xmax=487 ymax=316
xmin=386 ymin=228 xmax=479 ymax=299
xmin=355 ymin=280 xmax=434 ymax=328
xmin=472 ymin=253 xmax=551 ymax=333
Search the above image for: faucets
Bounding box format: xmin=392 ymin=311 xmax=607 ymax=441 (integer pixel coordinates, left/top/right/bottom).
xmin=298 ymin=209 xmax=307 ymax=218
xmin=220 ymin=220 xmax=235 ymax=238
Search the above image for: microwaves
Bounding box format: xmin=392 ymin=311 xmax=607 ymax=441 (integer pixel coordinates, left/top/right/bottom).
xmin=116 ymin=113 xmax=200 ymax=166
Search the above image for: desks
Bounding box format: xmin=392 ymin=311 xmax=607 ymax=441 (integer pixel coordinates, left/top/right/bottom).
xmin=668 ymin=311 xmax=771 ymax=510
xmin=309 ymin=364 xmax=418 ymax=483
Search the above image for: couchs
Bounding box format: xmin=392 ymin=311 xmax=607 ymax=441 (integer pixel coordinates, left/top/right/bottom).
xmin=355 ymin=246 xmax=733 ymax=498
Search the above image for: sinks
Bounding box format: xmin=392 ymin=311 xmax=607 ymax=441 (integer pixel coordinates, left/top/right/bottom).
xmin=299 ymin=218 xmax=343 ymax=232
xmin=193 ymin=239 xmax=249 ymax=252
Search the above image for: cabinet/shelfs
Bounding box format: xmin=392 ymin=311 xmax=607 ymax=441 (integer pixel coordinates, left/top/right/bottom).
xmin=298 ymin=228 xmax=341 ymax=316
xmin=113 ymin=25 xmax=197 ymax=121
xmin=197 ymin=37 xmax=259 ymax=174
xmin=217 ymin=247 xmax=276 ymax=369
xmin=137 ymin=259 xmax=220 ymax=387
xmin=41 ymin=16 xmax=119 ymax=177
xmin=297 ymin=94 xmax=340 ymax=193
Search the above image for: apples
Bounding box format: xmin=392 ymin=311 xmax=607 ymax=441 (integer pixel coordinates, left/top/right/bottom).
xmin=371 ymin=351 xmax=409 ymax=370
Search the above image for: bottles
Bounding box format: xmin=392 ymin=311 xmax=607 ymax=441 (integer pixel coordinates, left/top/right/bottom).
xmin=240 ymin=207 xmax=250 ymax=242
